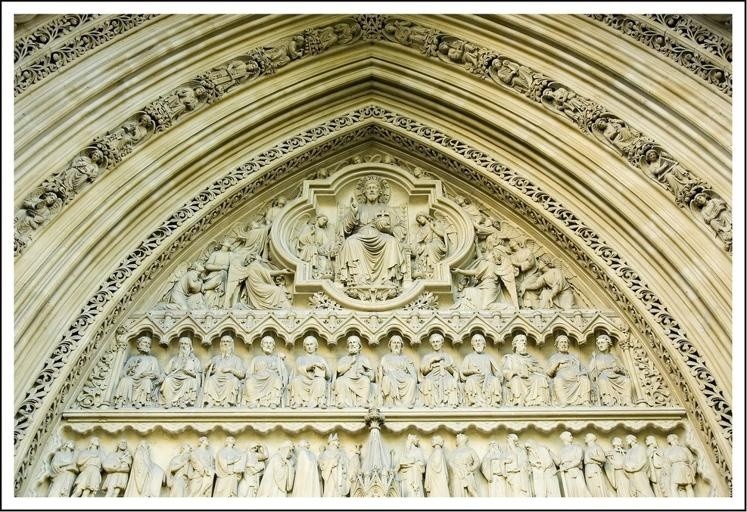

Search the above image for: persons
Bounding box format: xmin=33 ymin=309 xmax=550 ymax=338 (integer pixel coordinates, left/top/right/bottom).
xmin=646 ymin=434 xmax=697 ymax=497
xmin=162 ymin=178 xmax=654 ymax=496
xmin=49 ymin=336 xmax=159 ymax=496
xmin=14 ymin=20 xmax=731 ymax=251
